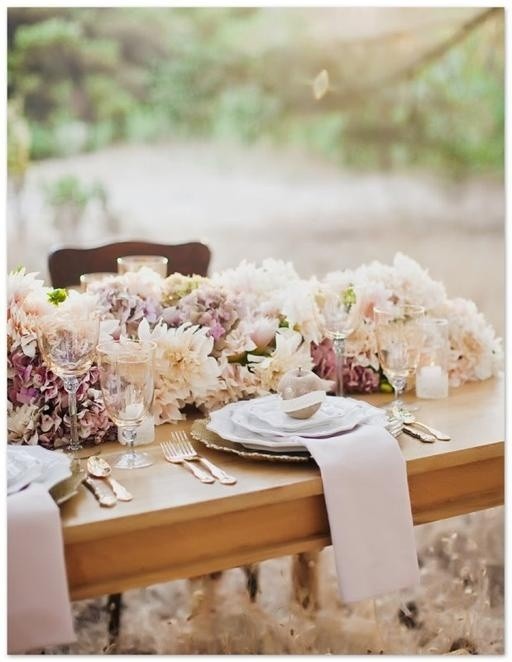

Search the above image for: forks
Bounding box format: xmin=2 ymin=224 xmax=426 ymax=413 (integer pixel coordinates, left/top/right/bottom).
xmin=159 ymin=428 xmax=238 ymax=486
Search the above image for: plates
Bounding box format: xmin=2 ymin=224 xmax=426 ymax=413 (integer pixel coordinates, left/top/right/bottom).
xmin=6 ymin=443 xmax=87 ymax=513
xmin=189 ymin=395 xmax=404 ymax=465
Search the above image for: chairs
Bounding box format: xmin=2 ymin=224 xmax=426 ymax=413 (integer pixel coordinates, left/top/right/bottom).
xmin=49 ymin=242 xmax=260 ymax=654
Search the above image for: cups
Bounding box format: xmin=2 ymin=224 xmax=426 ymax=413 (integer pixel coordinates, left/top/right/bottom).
xmin=412 ymin=317 xmax=452 ymax=400
xmin=79 ymin=254 xmax=170 ymax=291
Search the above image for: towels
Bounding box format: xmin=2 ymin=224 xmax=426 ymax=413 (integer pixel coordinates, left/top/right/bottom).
xmin=7 ymin=485 xmax=79 ymax=654
xmin=292 ymin=422 xmax=421 ymax=602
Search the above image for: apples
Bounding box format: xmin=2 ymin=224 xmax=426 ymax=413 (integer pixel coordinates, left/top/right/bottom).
xmin=278 ymin=365 xmax=325 ymax=418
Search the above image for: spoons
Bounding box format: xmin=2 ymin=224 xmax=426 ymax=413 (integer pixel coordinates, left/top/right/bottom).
xmin=391 ymin=403 xmax=452 ymax=444
xmin=86 ymin=455 xmax=133 ymax=504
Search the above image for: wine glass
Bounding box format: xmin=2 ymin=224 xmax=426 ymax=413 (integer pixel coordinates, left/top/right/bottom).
xmin=96 ymin=339 xmax=156 ymax=472
xmin=372 ymin=302 xmax=426 ymax=415
xmin=309 ymin=283 xmax=364 ymax=399
xmin=34 ymin=314 xmax=102 ymax=461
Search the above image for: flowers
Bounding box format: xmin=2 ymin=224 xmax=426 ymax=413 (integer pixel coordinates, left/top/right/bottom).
xmin=7 ymin=253 xmax=505 ymax=440
